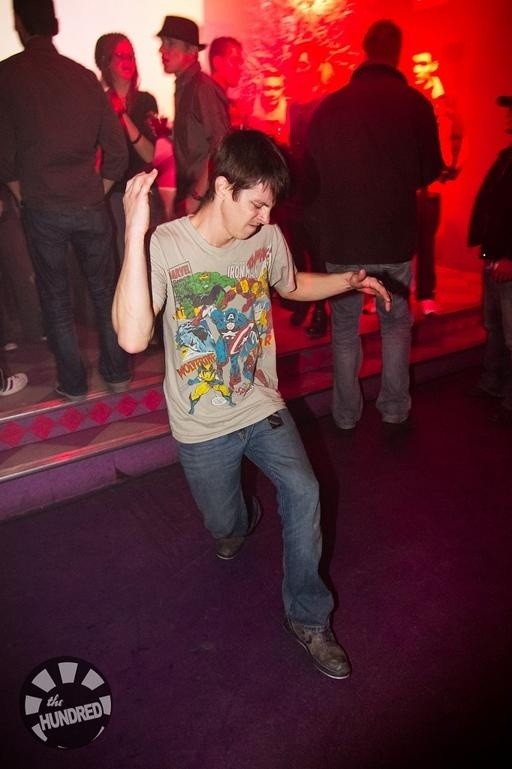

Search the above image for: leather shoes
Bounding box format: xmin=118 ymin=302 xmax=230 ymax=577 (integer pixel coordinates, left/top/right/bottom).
xmin=304 ymin=308 xmax=326 ymax=337
xmin=488 ymin=406 xmax=512 ymax=423
xmin=463 ymin=385 xmax=504 ymax=400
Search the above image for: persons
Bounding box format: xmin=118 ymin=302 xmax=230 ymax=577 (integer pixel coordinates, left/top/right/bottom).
xmin=112 ymin=128 xmax=392 ymax=680
xmin=300 ymin=15 xmax=444 ymax=437
xmin=1 ymin=0 xmax=512 ymax=401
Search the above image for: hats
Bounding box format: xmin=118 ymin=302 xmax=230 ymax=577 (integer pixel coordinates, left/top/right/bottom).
xmin=156 ymin=16 xmax=207 ymax=51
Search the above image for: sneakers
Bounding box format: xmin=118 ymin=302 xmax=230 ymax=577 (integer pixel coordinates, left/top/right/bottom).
xmin=107 ymin=365 xmax=134 ymax=394
xmin=3 ymin=342 xmax=18 ymax=351
xmin=282 ymin=613 xmax=352 ymax=680
xmin=216 ymin=495 xmax=262 ymax=561
xmin=54 ymin=379 xmax=86 ymax=401
xmin=0 ymin=372 xmax=28 ymax=396
